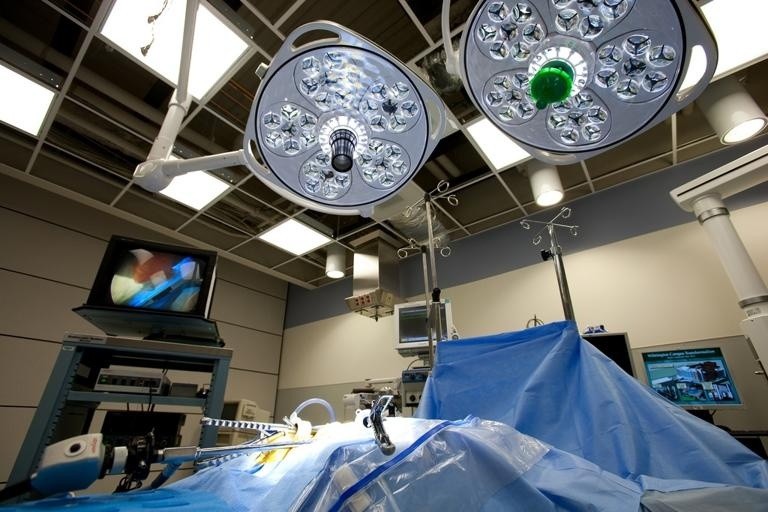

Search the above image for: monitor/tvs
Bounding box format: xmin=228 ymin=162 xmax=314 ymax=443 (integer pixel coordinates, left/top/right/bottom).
xmin=393 ymin=298 xmax=453 ymax=351
xmin=78 ymin=231 xmax=219 ymax=319
xmin=640 ymin=346 xmax=744 ymax=412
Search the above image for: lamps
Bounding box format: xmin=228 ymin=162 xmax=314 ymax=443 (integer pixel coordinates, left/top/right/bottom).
xmin=693 ymin=74 xmax=768 ymax=144
xmin=525 ymin=155 xmax=566 ymax=207
xmin=441 ymin=0 xmax=720 ymax=167
xmin=130 ymin=0 xmax=447 ymax=221
xmin=324 ymin=245 xmax=346 ymax=280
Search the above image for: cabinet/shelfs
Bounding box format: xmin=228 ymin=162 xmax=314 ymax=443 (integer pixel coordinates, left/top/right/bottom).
xmin=4 ymin=306 xmax=233 ymax=488
